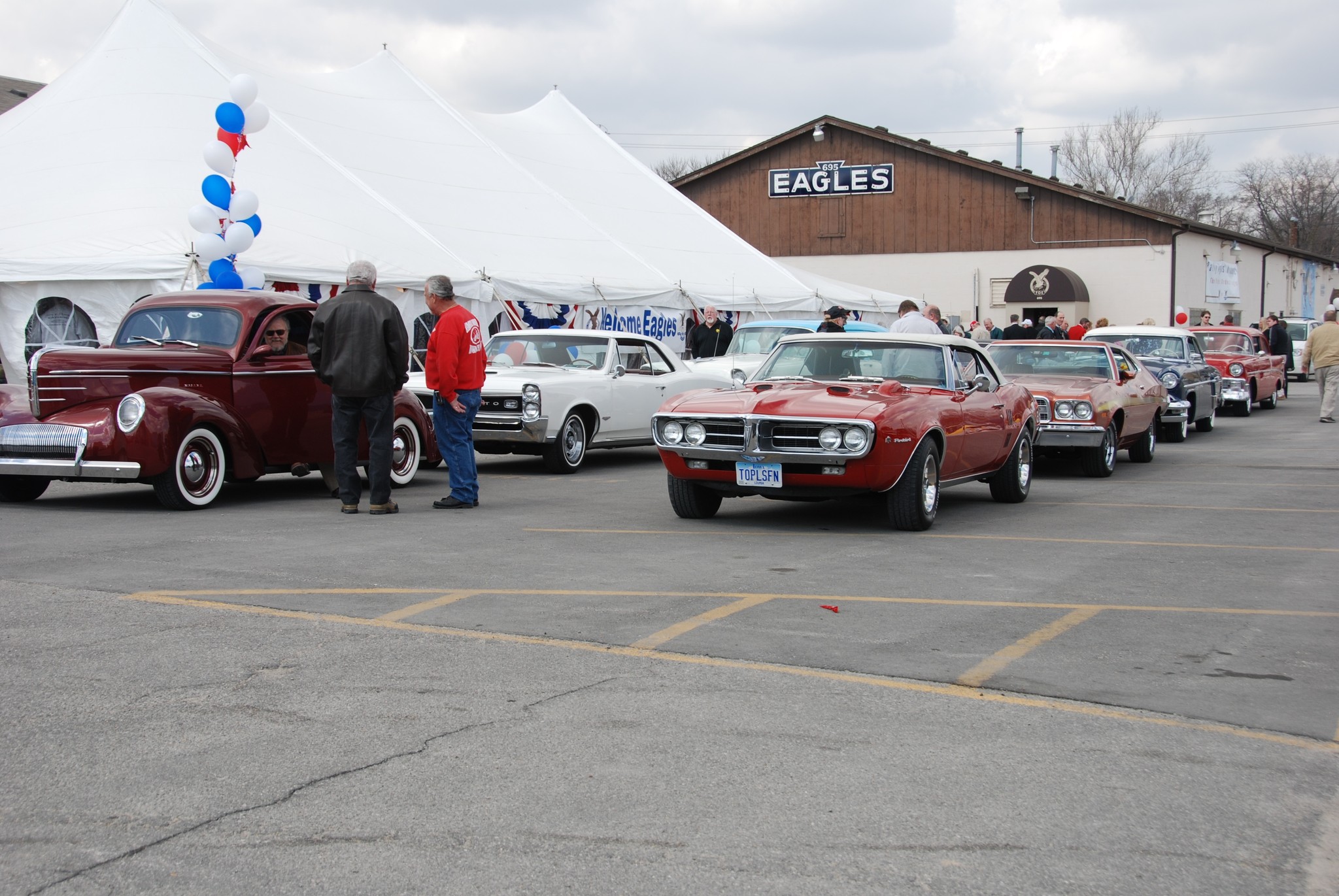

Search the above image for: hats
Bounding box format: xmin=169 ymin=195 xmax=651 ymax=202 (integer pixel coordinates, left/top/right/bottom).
xmin=970 ymin=321 xmax=980 ymax=331
xmin=1021 ymin=319 xmax=1032 ymax=325
xmin=824 ymin=305 xmax=851 ymax=320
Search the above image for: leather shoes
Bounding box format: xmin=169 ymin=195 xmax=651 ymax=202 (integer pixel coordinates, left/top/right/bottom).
xmin=433 ymin=494 xmax=479 ymax=509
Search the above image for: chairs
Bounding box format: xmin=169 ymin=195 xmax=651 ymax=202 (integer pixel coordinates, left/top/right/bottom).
xmin=1287 ymin=328 xmax=1306 ymax=339
xmin=1001 ymin=361 xmax=1127 ymax=378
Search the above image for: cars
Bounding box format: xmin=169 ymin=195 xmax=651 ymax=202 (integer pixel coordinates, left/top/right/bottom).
xmin=969 ymin=337 xmax=1002 ymax=349
xmin=1244 ymin=319 xmax=1324 ymax=382
xmin=643 ymin=319 xmax=891 ymax=387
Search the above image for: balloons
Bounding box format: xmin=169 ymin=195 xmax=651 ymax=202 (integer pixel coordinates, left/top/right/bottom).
xmin=739 ymin=339 xmax=760 ymax=353
xmin=492 ymin=326 xmax=578 ymax=366
xmin=1176 ymin=313 xmax=1188 ymax=324
xmin=1326 ymin=298 xmax=1339 ymax=311
xmin=1174 ymin=306 xmax=1184 ymax=317
xmin=188 ymin=74 xmax=265 ymax=290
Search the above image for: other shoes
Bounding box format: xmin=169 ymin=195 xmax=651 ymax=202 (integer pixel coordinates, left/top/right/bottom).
xmin=291 ymin=463 xmax=311 ymax=478
xmin=1319 ymin=416 xmax=1336 ymax=423
xmin=332 ymin=488 xmax=340 ymax=499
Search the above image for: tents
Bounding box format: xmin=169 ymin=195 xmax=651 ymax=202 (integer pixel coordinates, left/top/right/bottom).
xmin=0 ymin=0 xmax=925 ymax=384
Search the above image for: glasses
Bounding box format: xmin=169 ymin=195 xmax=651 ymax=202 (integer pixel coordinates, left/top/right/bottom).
xmin=266 ymin=329 xmax=287 ymax=336
xmin=1202 ymin=316 xmax=1211 ymax=319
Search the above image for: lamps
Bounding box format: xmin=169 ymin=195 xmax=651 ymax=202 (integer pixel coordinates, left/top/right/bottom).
xmin=1220 ymin=238 xmax=1242 ymax=257
xmin=812 ymin=123 xmax=829 ymax=143
xmin=1322 ymin=262 xmax=1338 ymax=273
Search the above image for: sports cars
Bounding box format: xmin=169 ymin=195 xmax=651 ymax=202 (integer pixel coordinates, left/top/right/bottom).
xmin=650 ymin=333 xmax=1042 ymax=532
xmin=1065 ymin=325 xmax=1223 ymax=444
xmin=1184 ymin=326 xmax=1286 ymax=417
xmin=964 ymin=339 xmax=1170 ymax=478
xmin=0 ymin=291 xmax=443 ymax=511
xmin=400 ymin=329 xmax=746 ymax=476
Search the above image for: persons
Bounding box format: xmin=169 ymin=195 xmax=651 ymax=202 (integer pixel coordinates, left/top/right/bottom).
xmin=264 ymin=316 xmax=340 ymax=499
xmin=1301 ymin=311 xmax=1339 ymax=423
xmin=1259 ymin=315 xmax=1295 ymax=400
xmin=882 ymin=300 xmax=944 ymax=378
xmin=424 ymin=275 xmax=487 ymax=508
xmin=691 ymin=305 xmax=740 ymax=360
xmin=804 ymin=306 xmax=862 ymax=376
xmin=942 ymin=319 xmax=951 ymax=334
xmin=921 ymin=304 xmax=950 ymax=378
xmin=1194 ymin=311 xmax=1244 ymax=352
xmin=970 ymin=321 xmax=991 ymax=339
xmin=1003 ymin=314 xmax=1163 ymax=355
xmin=983 ymin=318 xmax=1003 ymax=340
xmin=952 ymin=325 xmax=971 ymax=339
xmin=308 ymin=259 xmax=409 ymax=514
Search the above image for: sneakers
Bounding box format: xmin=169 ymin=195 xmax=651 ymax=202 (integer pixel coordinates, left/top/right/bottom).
xmin=340 ymin=503 xmax=358 ymax=513
xmin=369 ymin=499 xmax=400 ymax=514
